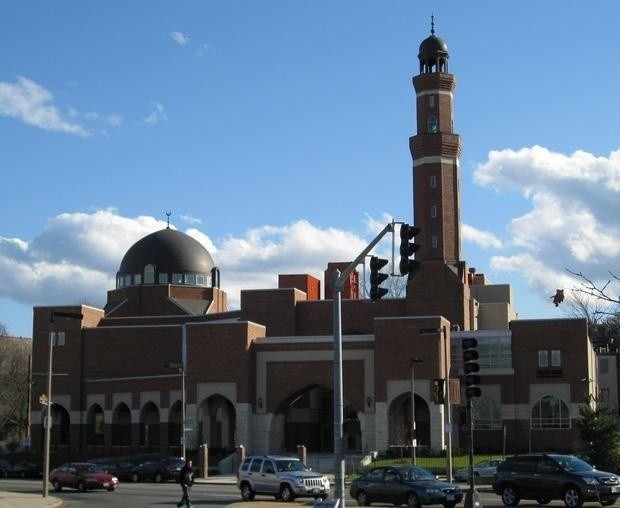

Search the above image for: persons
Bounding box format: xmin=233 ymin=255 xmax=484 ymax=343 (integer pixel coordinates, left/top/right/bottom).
xmin=176 ymin=459 xmax=195 ymax=508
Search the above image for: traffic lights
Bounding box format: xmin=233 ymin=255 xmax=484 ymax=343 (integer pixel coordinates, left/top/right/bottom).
xmin=370 ymin=256 xmax=389 ymax=299
xmin=400 ymin=224 xmax=422 ymax=275
xmin=462 ymin=336 xmax=482 ymax=398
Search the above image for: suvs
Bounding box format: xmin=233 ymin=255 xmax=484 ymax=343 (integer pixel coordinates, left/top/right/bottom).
xmin=237 ymin=454 xmax=330 ymax=502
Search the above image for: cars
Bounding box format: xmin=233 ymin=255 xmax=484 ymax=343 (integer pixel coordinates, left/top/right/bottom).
xmin=350 ymin=465 xmax=463 ymax=508
xmin=2 ymin=462 xmax=43 ymax=479
xmin=49 ymin=457 xmax=185 ymax=492
xmin=454 ymin=453 xmax=620 ymax=508
